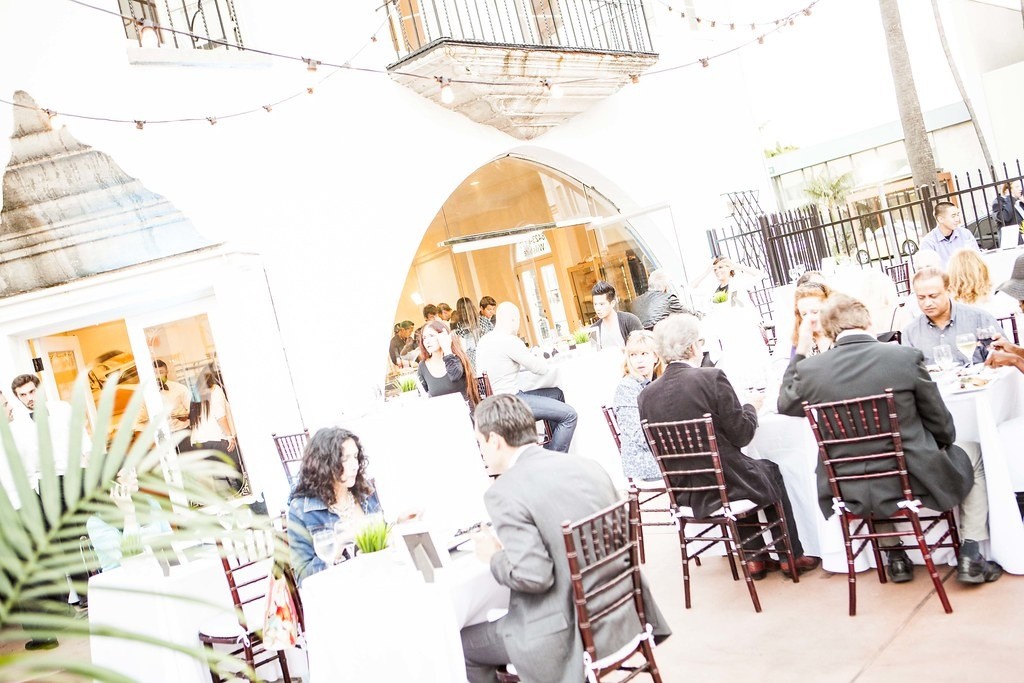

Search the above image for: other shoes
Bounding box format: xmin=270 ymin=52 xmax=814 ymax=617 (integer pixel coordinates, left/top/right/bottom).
xmin=79 ymin=598 xmax=88 ymax=608
xmin=25 ymin=637 xmax=59 ymax=650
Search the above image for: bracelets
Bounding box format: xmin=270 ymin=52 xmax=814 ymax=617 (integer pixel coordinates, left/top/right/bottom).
xmin=226 ymin=435 xmax=234 ymax=439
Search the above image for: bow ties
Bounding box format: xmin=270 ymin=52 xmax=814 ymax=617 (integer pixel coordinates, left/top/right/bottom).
xmin=160 ymin=384 xmax=169 ymax=390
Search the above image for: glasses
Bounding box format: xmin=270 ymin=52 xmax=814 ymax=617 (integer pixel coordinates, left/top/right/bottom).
xmin=695 ymin=338 xmax=705 ymax=346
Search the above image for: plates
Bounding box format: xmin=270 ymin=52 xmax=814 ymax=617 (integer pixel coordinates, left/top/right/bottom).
xmin=448 ymin=527 xmax=481 ymax=551
xmin=949 ymin=375 xmax=999 ymax=393
xmin=457 ymin=529 xmax=505 ymax=552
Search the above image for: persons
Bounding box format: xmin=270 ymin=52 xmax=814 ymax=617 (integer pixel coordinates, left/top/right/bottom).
xmin=135 ymin=360 xmax=244 ymax=492
xmin=460 ymin=393 xmax=673 ymax=683
xmin=477 ymin=302 xmax=578 ymax=454
xmin=0 ymin=374 xmax=93 ymax=649
xmin=550 ymin=293 xmax=569 ymax=337
xmin=389 ymin=296 xmax=497 ymax=413
xmin=777 ymin=270 xmax=1004 ymax=584
xmin=900 ymin=201 xmax=1024 ymax=371
xmin=589 ymin=254 xmax=820 ymax=578
xmin=288 ymin=427 xmax=426 ymax=589
xmin=993 ymin=180 xmax=1024 ymax=246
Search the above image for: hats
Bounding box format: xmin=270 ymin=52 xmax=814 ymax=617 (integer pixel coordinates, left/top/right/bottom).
xmin=995 ymin=254 xmax=1024 ymax=300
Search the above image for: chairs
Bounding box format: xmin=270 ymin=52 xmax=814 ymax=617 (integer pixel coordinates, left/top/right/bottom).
xmin=558 ymin=487 xmax=662 ymax=683
xmin=601 ymin=402 xmax=677 ymax=527
xmin=271 ymin=427 xmax=313 ymax=491
xmin=196 ymin=528 xmax=293 ymax=683
xmin=799 ymin=387 xmax=960 ymax=614
xmin=481 ymin=372 xmax=552 ymax=447
xmin=642 ymin=411 xmax=799 ymax=614
xmin=741 ymin=258 xmax=911 ymax=354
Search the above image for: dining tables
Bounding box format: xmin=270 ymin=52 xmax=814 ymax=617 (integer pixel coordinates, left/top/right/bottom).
xmin=87 ymin=537 xmax=266 ymax=683
xmin=292 ymin=509 xmax=507 ymax=682
xmin=728 ymin=365 xmax=1024 ymax=576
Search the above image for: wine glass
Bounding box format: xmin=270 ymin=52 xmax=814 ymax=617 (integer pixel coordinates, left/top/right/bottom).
xmin=933 ymin=345 xmax=953 ymax=373
xmin=314 ymin=517 xmax=358 ymax=568
xmin=978 ymin=325 xmax=1002 ymax=366
xmin=956 ymin=334 xmax=977 ymax=369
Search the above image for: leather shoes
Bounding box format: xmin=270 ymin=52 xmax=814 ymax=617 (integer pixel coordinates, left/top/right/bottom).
xmin=748 ymin=561 xmax=778 ymax=580
xmin=781 ymin=554 xmax=819 ymax=577
xmin=957 ymin=556 xmax=1003 ymax=583
xmin=888 ymin=555 xmax=914 ymax=581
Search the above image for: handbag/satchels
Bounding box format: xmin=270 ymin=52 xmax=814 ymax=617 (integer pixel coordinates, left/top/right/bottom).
xmin=263 ymin=570 xmax=298 ymax=651
